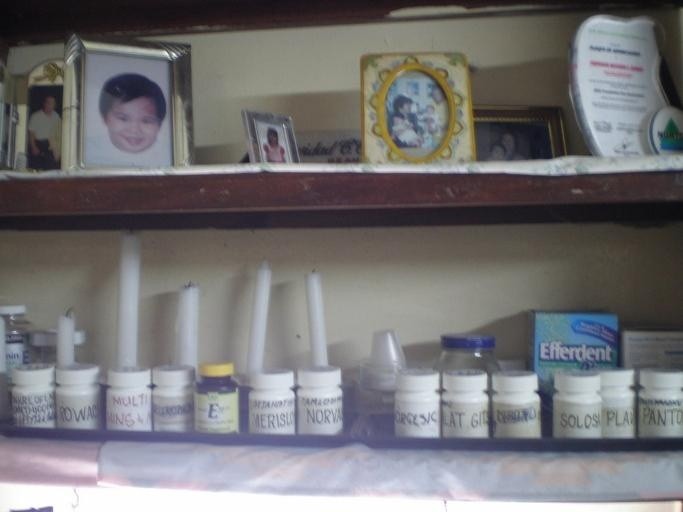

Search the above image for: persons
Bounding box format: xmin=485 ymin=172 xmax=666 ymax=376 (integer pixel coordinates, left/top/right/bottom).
xmin=262 ymin=128 xmax=286 ymax=162
xmin=487 ymin=141 xmax=508 ymax=161
xmin=98 ymin=72 xmax=167 ymax=154
xmin=28 ymin=92 xmax=64 ymax=170
xmin=392 ymin=93 xmax=438 ymax=150
xmin=501 ymin=132 xmax=525 ymax=160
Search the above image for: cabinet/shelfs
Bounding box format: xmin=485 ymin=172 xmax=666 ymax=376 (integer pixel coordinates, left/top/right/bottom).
xmin=1 ymin=0 xmax=683 ymax=455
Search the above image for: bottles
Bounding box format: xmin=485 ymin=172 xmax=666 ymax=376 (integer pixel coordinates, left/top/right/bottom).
xmin=553 ymin=372 xmax=602 ymax=439
xmin=437 ymin=336 xmax=499 ymax=378
xmin=192 ymin=361 xmax=240 ymax=432
xmin=639 ymin=370 xmax=683 ymax=436
xmin=1 ymin=303 xmax=33 ymax=402
xmin=492 ymin=372 xmax=541 ymax=438
xmin=106 ymin=365 xmax=153 ymax=433
xmin=599 ymin=371 xmax=635 ymax=438
xmin=11 ymin=362 xmax=55 ymax=431
xmin=247 ymin=368 xmax=295 ymax=434
xmin=55 ymin=361 xmax=103 ymax=432
xmin=440 ymin=369 xmax=489 ymax=437
xmin=295 ymin=364 xmax=344 ymax=432
xmin=394 ymin=368 xmax=442 ymax=438
xmin=151 ymin=364 xmax=195 ymax=433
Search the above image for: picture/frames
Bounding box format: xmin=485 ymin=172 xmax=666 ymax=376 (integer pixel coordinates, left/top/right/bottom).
xmin=6 ymin=33 xmax=194 ymax=171
xmin=240 ymin=109 xmax=300 ymax=164
xmin=357 ymin=51 xmax=568 ymax=166
